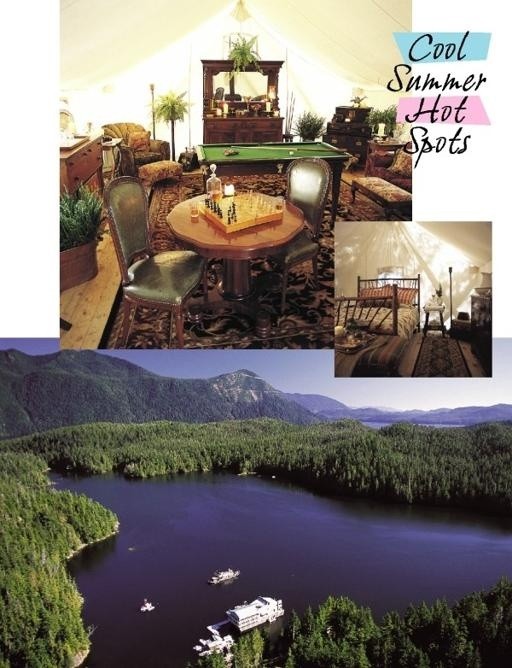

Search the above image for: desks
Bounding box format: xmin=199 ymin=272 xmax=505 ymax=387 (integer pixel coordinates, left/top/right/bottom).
xmin=196 ymin=141 xmax=352 ymax=230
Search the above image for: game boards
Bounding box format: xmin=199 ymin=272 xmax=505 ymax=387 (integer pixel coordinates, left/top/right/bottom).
xmin=198 ymin=193 xmax=283 ymax=234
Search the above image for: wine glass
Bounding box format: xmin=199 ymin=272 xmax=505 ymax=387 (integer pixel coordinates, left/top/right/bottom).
xmin=243 ymin=95 xmax=251 ymax=113
xmin=228 ymin=108 xmax=235 ymax=114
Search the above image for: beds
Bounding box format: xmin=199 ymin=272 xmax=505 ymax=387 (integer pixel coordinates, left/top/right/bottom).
xmin=334 ymin=272 xmax=419 ymax=345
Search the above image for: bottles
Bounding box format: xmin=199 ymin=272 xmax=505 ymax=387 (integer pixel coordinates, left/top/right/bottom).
xmin=206 ymin=164 xmax=223 ymax=200
xmin=210 ymin=97 xmax=216 ymax=110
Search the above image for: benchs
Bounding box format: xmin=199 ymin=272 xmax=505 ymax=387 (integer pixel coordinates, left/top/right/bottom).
xmin=352 ymin=176 xmax=411 ymax=220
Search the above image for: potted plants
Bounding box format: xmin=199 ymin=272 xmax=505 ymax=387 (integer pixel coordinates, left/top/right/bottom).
xmin=60 ymin=181 xmax=111 ymax=293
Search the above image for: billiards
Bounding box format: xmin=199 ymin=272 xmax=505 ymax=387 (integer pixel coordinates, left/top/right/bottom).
xmin=224 ymin=148 xmax=238 ymax=155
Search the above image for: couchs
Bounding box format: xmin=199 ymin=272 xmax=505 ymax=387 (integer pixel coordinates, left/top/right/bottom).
xmin=367 ymin=142 xmax=412 ymax=191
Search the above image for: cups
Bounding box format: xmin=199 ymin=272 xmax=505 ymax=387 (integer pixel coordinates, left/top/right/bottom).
xmin=275 ymin=200 xmax=285 ymax=211
xmin=251 ymin=105 xmax=260 ymax=112
xmin=189 ymin=201 xmax=199 ymax=217
xmin=237 ymin=111 xmax=245 ymax=116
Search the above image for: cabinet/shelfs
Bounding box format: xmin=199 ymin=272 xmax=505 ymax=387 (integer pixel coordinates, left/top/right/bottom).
xmin=60 ymin=134 xmax=103 ymax=195
xmin=201 ymin=60 xmax=285 ymax=143
xmin=321 ymin=105 xmax=376 ymax=165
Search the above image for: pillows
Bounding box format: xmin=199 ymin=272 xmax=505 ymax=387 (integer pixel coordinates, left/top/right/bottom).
xmin=386 ymin=149 xmax=411 ymax=176
xmin=358 ymin=284 xmax=419 ymax=308
xmin=126 ymin=130 xmax=150 ymax=153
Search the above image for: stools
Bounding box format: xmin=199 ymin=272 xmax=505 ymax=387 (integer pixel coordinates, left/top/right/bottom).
xmin=423 ymin=303 xmax=445 ymax=338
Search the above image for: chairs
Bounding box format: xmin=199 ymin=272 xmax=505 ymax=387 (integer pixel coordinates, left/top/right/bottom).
xmin=101 ymin=123 xmax=170 ymax=177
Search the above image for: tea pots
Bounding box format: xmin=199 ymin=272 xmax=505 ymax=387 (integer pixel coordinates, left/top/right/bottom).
xmin=480 ymin=272 xmax=491 ymax=289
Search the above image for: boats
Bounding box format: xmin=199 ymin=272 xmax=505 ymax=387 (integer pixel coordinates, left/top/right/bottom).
xmin=140 ymin=597 xmax=156 ymax=613
xmin=206 ymin=566 xmax=239 ymax=585
xmin=225 ymin=594 xmax=285 ymax=632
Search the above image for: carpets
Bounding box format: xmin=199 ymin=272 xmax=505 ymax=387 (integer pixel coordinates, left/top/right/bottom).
xmin=96 ymin=164 xmax=411 ymax=348
xmin=410 ymin=332 xmax=471 ymax=376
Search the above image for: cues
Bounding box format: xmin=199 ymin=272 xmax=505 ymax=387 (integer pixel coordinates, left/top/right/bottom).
xmin=285 ymin=49 xmax=295 ymax=141
xmin=231 ymin=146 xmax=347 ymax=152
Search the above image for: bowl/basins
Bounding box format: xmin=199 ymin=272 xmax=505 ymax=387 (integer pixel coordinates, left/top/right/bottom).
xmin=474 ymin=288 xmax=490 ymax=298
